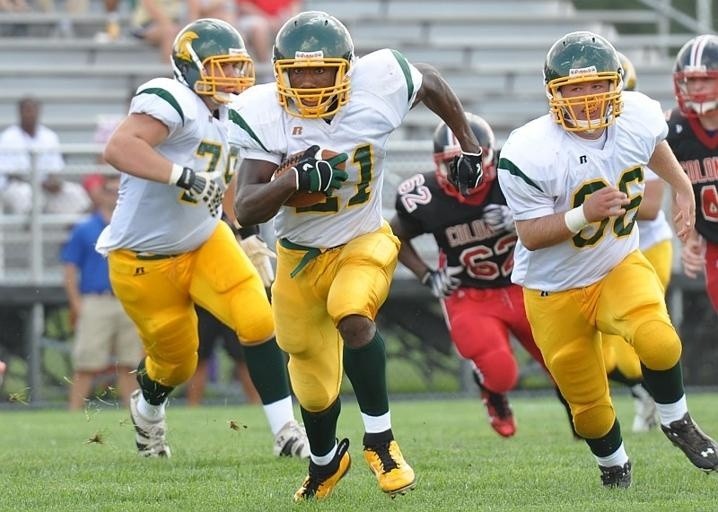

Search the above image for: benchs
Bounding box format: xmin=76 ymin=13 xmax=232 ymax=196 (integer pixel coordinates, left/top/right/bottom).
xmin=0 ymin=0 xmax=718 ymax=265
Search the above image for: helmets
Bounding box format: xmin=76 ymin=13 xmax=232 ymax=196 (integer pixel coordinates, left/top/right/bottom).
xmin=269 ymin=7 xmax=357 ymax=100
xmin=540 ymin=27 xmax=624 ymax=118
xmin=429 ymin=109 xmax=498 ymax=191
xmin=670 ymin=32 xmax=718 ymax=114
xmin=167 ymin=16 xmax=253 ymax=94
xmin=611 ymin=48 xmax=640 ymax=92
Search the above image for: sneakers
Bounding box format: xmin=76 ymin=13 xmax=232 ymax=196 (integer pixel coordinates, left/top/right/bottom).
xmin=127 ymin=388 xmax=173 ymax=459
xmin=655 ymin=409 xmax=718 ymax=474
xmin=360 ymin=425 xmax=419 ymax=500
xmin=596 ymin=458 xmax=634 ymax=489
xmin=272 ymin=418 xmax=340 ymax=462
xmin=470 ymin=364 xmax=518 ymax=439
xmin=292 ymin=433 xmax=353 ymax=504
xmin=630 ymin=386 xmax=662 ymax=436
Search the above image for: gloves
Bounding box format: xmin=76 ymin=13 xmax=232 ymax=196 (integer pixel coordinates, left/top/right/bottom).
xmin=236 ymin=224 xmax=279 ymax=289
xmin=168 ymin=163 xmax=225 ymax=218
xmin=444 ymin=147 xmax=488 ymax=199
xmin=419 ymin=261 xmax=468 ymax=299
xmin=288 ymin=143 xmax=352 ymax=199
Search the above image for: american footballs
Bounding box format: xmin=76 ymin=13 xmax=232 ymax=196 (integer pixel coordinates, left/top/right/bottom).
xmin=270 ymin=149 xmax=346 ymax=208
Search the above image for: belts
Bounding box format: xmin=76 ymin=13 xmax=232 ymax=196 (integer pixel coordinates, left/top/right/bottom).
xmin=280 ymin=236 xmax=349 ymax=279
